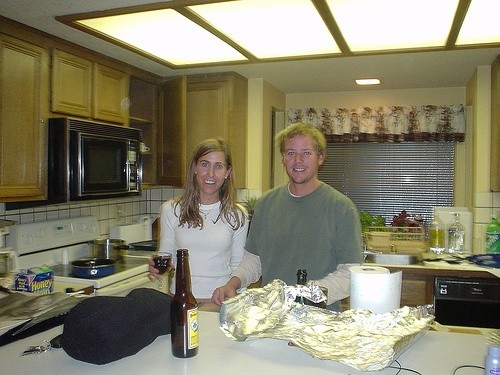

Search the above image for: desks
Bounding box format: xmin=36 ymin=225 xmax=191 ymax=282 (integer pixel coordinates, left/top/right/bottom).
xmin=0 ymin=294 xmax=500 ymax=375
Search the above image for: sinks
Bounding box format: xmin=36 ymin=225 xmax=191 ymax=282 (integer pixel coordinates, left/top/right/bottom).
xmin=362 ymin=252 xmax=423 ymax=266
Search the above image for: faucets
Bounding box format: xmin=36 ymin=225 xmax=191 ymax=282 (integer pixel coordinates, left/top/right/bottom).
xmin=361 ymin=242 xmax=367 ymax=254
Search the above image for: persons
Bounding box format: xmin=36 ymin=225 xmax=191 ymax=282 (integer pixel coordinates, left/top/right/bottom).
xmin=210 ymin=122 xmax=363 ymax=313
xmin=147 ymin=138 xmax=248 ymax=298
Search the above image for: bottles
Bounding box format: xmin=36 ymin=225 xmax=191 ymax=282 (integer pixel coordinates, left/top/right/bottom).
xmin=170 ymin=248 xmax=199 ymax=358
xmin=485 ymin=344 xmax=499 ymax=375
xmin=486 ymin=216 xmax=500 ymax=256
xmin=429 ymin=211 xmax=445 ymax=255
xmin=447 ymin=213 xmax=466 ymax=255
xmin=294 ymin=268 xmax=311 ymax=307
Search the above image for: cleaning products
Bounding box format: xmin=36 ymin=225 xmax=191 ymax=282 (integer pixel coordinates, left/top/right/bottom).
xmin=429 ymin=212 xmax=446 ymax=255
xmin=485 ymin=217 xmax=500 ymax=254
xmin=448 ymin=213 xmax=466 ymax=254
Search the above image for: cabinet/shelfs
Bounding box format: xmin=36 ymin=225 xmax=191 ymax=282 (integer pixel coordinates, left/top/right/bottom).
xmin=0 ymin=14 xmax=248 ymax=204
xmin=340 ymin=268 xmax=433 ymax=312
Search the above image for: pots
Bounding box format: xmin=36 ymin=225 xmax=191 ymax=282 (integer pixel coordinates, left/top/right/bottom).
xmin=71 ymin=257 xmax=116 ymax=279
xmin=89 ymin=237 xmax=133 ymax=262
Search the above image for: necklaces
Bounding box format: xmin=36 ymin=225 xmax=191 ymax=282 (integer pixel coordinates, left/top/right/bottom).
xmin=199 ymin=201 xmax=221 ymax=220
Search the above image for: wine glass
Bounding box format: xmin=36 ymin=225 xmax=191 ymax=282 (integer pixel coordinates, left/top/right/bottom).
xmin=151 ymin=251 xmax=172 ymax=292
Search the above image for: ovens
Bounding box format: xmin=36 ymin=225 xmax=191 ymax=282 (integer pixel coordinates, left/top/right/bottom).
xmin=432 ymin=275 xmax=500 ymax=333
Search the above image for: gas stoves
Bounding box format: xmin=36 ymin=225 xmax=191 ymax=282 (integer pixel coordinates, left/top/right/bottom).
xmin=7 ymin=215 xmax=154 ymax=297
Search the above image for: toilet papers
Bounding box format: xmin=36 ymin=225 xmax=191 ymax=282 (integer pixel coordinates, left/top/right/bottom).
xmin=348 ymin=264 xmax=403 ymax=313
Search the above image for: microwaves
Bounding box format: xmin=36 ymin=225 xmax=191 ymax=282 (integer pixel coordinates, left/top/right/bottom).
xmin=48 ymin=116 xmax=143 ymax=203
